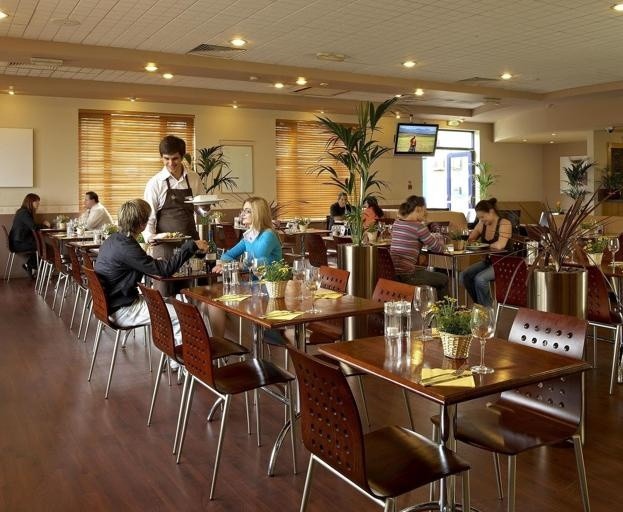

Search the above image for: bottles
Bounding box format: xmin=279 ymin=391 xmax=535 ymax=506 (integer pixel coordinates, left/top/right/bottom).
xmin=384 ymin=301 xmax=410 ymax=339
xmin=384 ymin=339 xmax=411 ymax=372
xmin=223 ymin=285 xmax=240 ymax=306
xmin=206 ymin=230 xmax=217 ymax=274
xmin=223 ymin=262 xmax=241 ymax=286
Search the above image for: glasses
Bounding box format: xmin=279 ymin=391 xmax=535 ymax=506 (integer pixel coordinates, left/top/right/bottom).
xmin=241 ymin=208 xmax=252 ymax=214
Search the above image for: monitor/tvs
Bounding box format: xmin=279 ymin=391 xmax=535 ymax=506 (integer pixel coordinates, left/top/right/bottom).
xmin=393 ymin=122 xmax=440 ymax=157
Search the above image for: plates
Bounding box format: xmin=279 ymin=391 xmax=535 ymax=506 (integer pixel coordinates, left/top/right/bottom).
xmin=184 ymin=199 xmax=224 ymax=205
xmin=466 ymin=243 xmax=490 ymax=250
xmin=151 ymin=236 xmax=192 ymax=242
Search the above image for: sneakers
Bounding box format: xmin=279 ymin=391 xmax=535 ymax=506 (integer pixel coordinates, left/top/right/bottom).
xmin=22 ymin=263 xmax=33 ymax=279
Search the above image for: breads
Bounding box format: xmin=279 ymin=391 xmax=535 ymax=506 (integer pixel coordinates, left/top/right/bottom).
xmin=193 ymin=195 xmax=218 ymax=201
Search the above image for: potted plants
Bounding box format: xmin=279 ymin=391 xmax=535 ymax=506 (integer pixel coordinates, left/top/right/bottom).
xmin=183 ymin=146 xmax=239 ymax=242
xmin=305 ymin=97 xmax=416 ymax=342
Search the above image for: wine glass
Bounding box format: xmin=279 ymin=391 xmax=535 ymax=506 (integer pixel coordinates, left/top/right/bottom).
xmin=414 ymin=286 xmax=434 ymax=341
xmin=541 ymin=233 xmax=619 ymax=267
xmin=243 ymin=251 xmax=322 ymax=313
xmin=344 ymin=220 xmax=469 ymax=252
xmin=470 ymin=305 xmax=496 ymax=374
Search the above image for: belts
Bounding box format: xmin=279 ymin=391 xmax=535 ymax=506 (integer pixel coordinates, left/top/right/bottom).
xmin=108 ymin=307 xmax=121 ymax=316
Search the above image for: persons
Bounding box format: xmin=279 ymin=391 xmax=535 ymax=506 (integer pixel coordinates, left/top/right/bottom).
xmin=328 ymin=193 xmax=357 ymax=237
xmin=409 ymin=135 xmax=417 ymax=152
xmin=94 ymin=198 xmax=209 ymax=373
xmin=201 ymin=196 xmax=282 ymax=340
xmin=460 ymin=198 xmax=514 ymax=309
xmin=390 ymin=196 xmax=448 ymax=304
xmin=359 ymin=196 xmax=386 ymax=233
xmin=143 ymin=134 xmax=214 ymax=298
xmin=9 ymin=193 xmax=50 ymax=281
xmin=73 ymin=191 xmax=115 ymax=234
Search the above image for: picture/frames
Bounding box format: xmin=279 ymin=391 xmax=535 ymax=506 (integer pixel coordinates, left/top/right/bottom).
xmin=607 ymin=142 xmax=623 ymax=187
